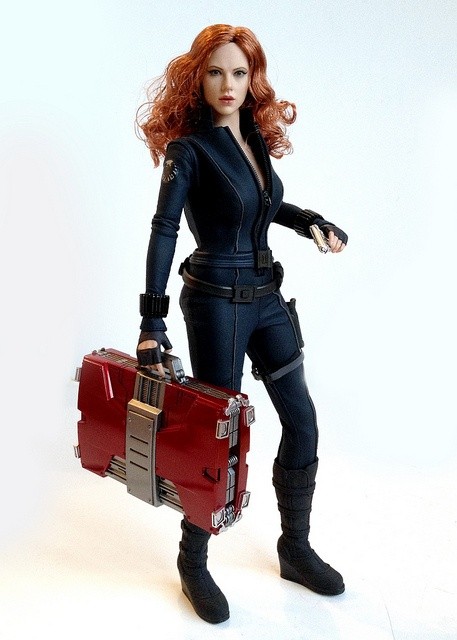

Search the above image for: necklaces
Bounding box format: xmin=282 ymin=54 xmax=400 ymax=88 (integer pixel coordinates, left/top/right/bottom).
xmin=235 ymin=136 xmax=253 ymax=156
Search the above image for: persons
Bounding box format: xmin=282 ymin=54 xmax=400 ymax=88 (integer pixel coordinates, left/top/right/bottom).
xmin=133 ymin=24 xmax=349 ymax=623
